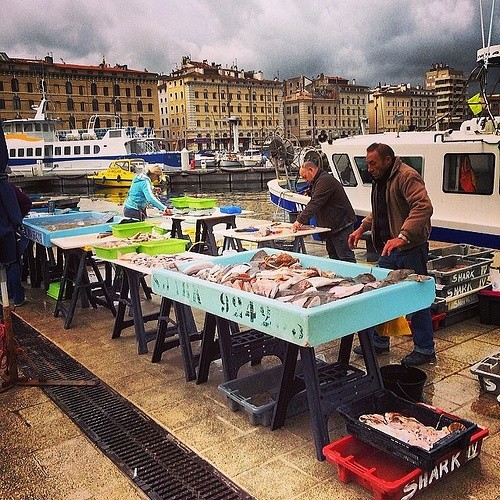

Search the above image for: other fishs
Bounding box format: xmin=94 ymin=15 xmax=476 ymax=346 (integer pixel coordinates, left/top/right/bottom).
xmin=259 ymin=226 xmax=270 ymax=237
xmin=184 ymin=248 xmax=432 ymax=308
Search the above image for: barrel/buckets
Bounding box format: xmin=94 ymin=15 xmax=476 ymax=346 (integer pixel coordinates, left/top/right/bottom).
xmin=379 ymin=361 xmax=428 ymax=404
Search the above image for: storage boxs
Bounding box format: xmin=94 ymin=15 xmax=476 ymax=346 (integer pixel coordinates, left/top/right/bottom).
xmin=467 ymin=351 xmax=500 ymax=395
xmin=169 ymin=196 xmax=192 ymax=207
xmin=187 ymin=198 xmax=220 ymax=209
xmin=140 ymin=238 xmax=192 ymax=256
xmin=107 ymin=222 xmax=156 ymax=238
xmin=320 ymin=401 xmax=491 ymax=500
xmin=46 ymin=282 xmax=64 ymax=300
xmin=216 ymin=354 xmax=338 ymax=425
xmin=424 ymin=242 xmax=496 ymax=320
xmin=334 ymin=387 xmax=480 ymax=471
xmin=91 ymin=242 xmax=141 ymax=259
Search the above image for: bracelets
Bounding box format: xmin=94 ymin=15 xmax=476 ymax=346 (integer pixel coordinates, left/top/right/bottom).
xmin=398 ymin=233 xmax=408 ymax=242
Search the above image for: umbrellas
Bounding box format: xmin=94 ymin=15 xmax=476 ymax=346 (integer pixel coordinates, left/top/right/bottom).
xmin=0 ymin=115 xmax=29 ymax=383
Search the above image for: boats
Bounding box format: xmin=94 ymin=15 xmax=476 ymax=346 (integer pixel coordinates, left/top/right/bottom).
xmin=2 ymin=75 xmax=197 ymax=175
xmin=267 ymin=1 xmax=500 ymax=250
xmin=192 ymin=146 xmax=316 ymax=174
xmin=84 ymin=154 xmax=171 ymax=189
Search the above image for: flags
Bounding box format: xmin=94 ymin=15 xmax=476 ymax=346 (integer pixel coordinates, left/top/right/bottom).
xmin=468 ymin=96 xmax=482 ymax=115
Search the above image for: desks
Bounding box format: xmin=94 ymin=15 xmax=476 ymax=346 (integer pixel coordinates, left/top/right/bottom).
xmin=161 ymin=209 xmax=253 ymax=252
xmin=212 ymin=222 xmax=332 ymax=255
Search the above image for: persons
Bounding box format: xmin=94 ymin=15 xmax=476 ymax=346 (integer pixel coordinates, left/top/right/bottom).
xmin=348 ymin=142 xmax=436 ymax=367
xmin=123 ymin=165 xmax=172 ymax=221
xmin=6 ymin=181 xmax=32 ymax=307
xmin=292 ymin=161 xmax=358 ymax=264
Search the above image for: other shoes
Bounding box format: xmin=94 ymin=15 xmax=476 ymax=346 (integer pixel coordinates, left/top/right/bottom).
xmin=148 ymin=165 xmax=161 ymax=174
xmin=401 ymin=348 xmax=436 ymax=367
xmin=14 ymin=297 xmax=27 ymax=307
xmin=353 ymin=336 xmax=391 ymax=356
